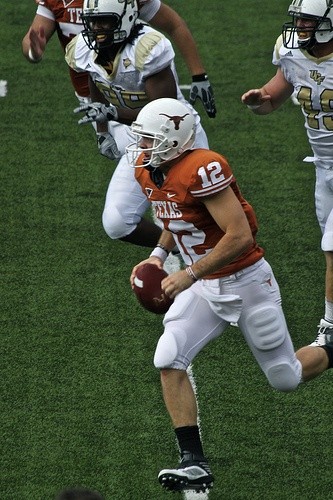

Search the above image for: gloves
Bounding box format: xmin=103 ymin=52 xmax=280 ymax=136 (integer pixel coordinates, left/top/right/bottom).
xmin=73 ymin=102 xmax=118 ymax=121
xmin=190 ymin=72 xmax=218 ymax=118
xmin=97 ymin=132 xmax=121 ymax=161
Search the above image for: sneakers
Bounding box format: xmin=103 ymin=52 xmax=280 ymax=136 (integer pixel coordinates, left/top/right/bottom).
xmin=159 ymin=450 xmax=213 ymax=494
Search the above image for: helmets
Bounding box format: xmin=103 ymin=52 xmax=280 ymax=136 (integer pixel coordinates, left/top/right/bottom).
xmin=80 ymin=0 xmax=138 ymax=55
xmin=127 ymin=97 xmax=196 ymax=168
xmin=282 ymin=0 xmax=332 ymax=49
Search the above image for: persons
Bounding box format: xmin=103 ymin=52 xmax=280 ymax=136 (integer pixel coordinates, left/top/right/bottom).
xmin=65 ymin=0 xmax=210 ymax=254
xmin=242 ymin=0 xmax=333 ymax=346
xmin=124 ymin=97 xmax=333 ymax=495
xmin=22 ymin=0 xmax=217 ymax=133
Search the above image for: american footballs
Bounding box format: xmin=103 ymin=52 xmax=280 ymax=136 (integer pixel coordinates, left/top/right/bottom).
xmin=133 ymin=262 xmax=175 ymax=314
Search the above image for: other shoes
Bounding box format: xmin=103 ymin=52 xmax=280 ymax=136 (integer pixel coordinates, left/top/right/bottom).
xmin=307 ymin=318 xmax=333 ymax=348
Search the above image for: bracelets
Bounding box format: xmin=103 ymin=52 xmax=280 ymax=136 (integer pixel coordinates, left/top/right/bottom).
xmin=185 ymin=267 xmax=198 ymax=282
xmin=150 ymin=247 xmax=168 ymax=262
xmin=156 ymin=244 xmax=169 ymax=255
xmin=247 ymin=105 xmax=260 ymax=110
